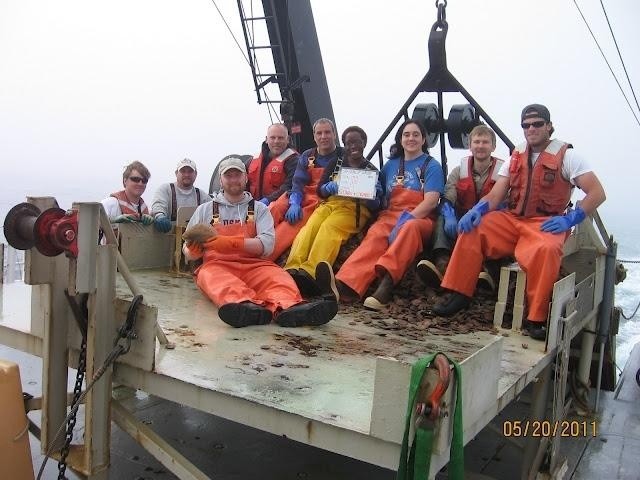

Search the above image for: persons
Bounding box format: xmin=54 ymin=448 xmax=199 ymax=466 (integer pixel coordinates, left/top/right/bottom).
xmin=150 ymin=158 xmax=214 ymax=234
xmin=281 ymin=126 xmax=387 ymax=297
xmin=417 ymin=124 xmax=506 ymax=297
xmin=97 ymin=161 xmax=155 ymax=246
xmin=246 ymin=122 xmax=299 ymax=207
xmin=183 ymin=157 xmax=338 ymax=328
xmin=315 ymin=118 xmax=445 ymax=310
xmin=431 ymin=104 xmax=607 ymax=342
xmin=262 ymin=117 xmax=346 ymax=263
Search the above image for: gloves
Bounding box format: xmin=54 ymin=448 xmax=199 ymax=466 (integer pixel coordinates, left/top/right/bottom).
xmin=109 ymin=214 xmax=172 ymax=233
xmin=186 ymin=234 xmax=244 ymax=260
xmin=388 ymin=209 xmax=417 ymax=247
xmin=320 ymin=181 xmax=339 ymax=198
xmin=366 ymin=181 xmax=383 ymax=211
xmin=539 ymin=207 xmax=585 ymax=234
xmin=284 ymin=192 xmax=303 ymax=225
xmin=441 ymin=201 xmax=488 ymax=240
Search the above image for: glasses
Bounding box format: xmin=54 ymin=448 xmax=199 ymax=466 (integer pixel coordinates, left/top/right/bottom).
xmin=521 ymin=121 xmax=547 ymax=128
xmin=129 ymin=176 xmax=148 ymax=184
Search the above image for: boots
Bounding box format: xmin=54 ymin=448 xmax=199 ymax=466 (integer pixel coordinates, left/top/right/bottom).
xmin=416 ymin=254 xmax=499 ymax=317
xmin=522 ymin=315 xmax=546 ymax=340
xmin=362 ymin=269 xmax=393 ymax=310
xmin=286 ymin=261 xmax=352 ymax=304
xmin=218 ymin=301 xmax=338 ymax=327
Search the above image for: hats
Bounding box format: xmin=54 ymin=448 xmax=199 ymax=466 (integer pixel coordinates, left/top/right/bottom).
xmin=219 ymin=158 xmax=246 ymax=178
xmin=521 ymin=104 xmax=554 ymax=136
xmin=177 ymin=159 xmax=197 ymax=171
